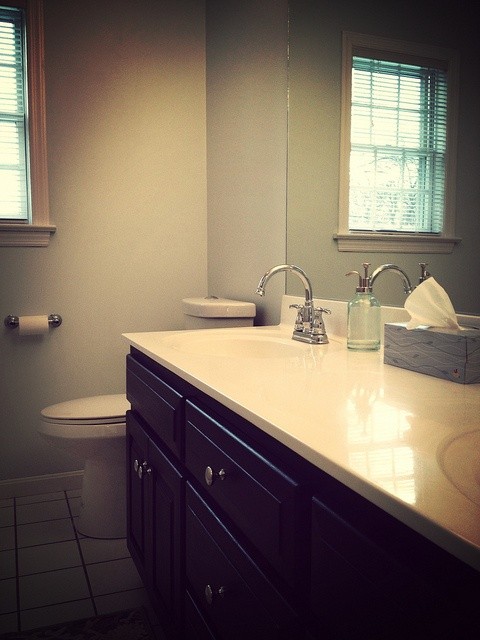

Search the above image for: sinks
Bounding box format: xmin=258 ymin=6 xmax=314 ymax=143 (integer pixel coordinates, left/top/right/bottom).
xmin=162 ymin=328 xmax=312 ymax=361
xmin=436 ymin=429 xmax=480 ymax=507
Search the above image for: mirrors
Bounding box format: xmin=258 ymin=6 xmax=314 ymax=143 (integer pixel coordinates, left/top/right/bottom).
xmin=283 ymin=2 xmax=479 ymax=319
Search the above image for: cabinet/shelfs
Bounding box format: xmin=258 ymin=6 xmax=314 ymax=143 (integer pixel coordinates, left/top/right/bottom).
xmin=304 ymin=480 xmax=480 ymax=640
xmin=179 ymin=395 xmax=304 ymax=639
xmin=126 ymin=346 xmax=181 ymax=639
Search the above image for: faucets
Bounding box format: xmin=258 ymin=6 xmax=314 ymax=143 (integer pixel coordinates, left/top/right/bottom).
xmin=369 ymin=262 xmax=430 ymax=296
xmin=256 ymin=265 xmax=331 ymax=344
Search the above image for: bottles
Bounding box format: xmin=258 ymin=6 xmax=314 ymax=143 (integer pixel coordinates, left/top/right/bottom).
xmin=347 ymin=286 xmax=379 ymax=350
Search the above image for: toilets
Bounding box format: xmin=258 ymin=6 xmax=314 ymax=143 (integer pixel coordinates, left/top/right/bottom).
xmin=36 ymin=296 xmax=256 ymax=540
